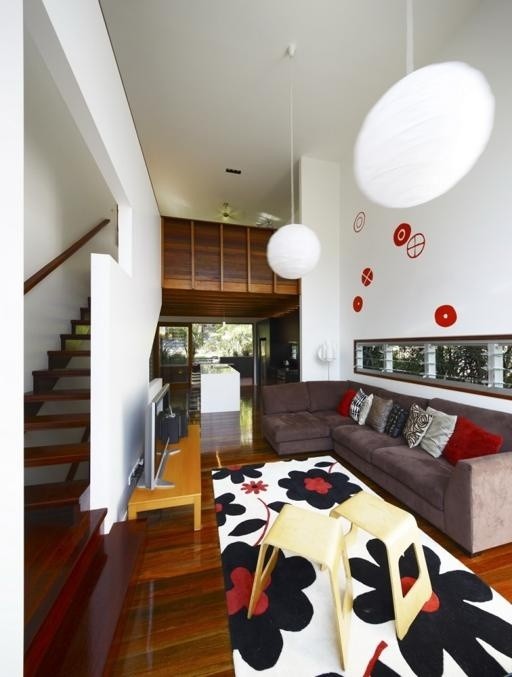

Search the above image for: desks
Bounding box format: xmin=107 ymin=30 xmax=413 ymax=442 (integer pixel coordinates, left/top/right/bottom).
xmin=126 ymin=425 xmax=202 ymax=531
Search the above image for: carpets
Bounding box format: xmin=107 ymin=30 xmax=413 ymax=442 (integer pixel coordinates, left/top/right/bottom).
xmin=211 ymin=455 xmax=512 ymax=677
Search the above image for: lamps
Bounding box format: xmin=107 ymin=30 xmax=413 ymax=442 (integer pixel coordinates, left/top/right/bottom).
xmin=318 ymin=339 xmax=337 ymax=381
xmin=267 ymin=43 xmax=320 ymax=280
xmin=352 ymin=0 xmax=496 ymax=209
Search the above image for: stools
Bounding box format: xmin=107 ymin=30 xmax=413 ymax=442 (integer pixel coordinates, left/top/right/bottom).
xmin=320 ymin=491 xmax=433 ymax=641
xmin=247 ymin=503 xmax=354 ymax=672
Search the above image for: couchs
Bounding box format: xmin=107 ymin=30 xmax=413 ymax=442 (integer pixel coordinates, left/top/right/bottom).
xmin=261 ymin=380 xmax=512 ymax=558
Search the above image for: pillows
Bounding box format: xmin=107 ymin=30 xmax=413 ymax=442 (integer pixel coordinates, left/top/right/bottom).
xmin=459 ymin=404 xmax=512 ymax=452
xmin=390 ymin=410 xmax=408 ymax=438
xmin=403 ymin=402 xmax=433 ymax=448
xmin=419 ymin=406 xmax=458 ymax=458
xmin=336 ymin=387 xmax=357 ymax=416
xmin=366 ymin=395 xmax=394 ymax=434
xmin=262 ymin=382 xmax=310 ymax=415
xmin=358 ymin=393 xmax=374 ymax=425
xmin=442 ymin=415 xmax=505 ymax=467
xmin=349 ymin=387 xmax=368 ymax=421
xmin=346 ymin=380 xmax=384 ymax=400
xmin=305 ymin=381 xmax=350 ymax=413
xmin=384 ymin=400 xmax=404 ymax=435
xmin=378 ymin=391 xmax=428 ymax=411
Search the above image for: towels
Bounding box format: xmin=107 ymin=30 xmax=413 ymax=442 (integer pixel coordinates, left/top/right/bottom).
xmin=428 ymin=397 xmax=464 ymax=419
